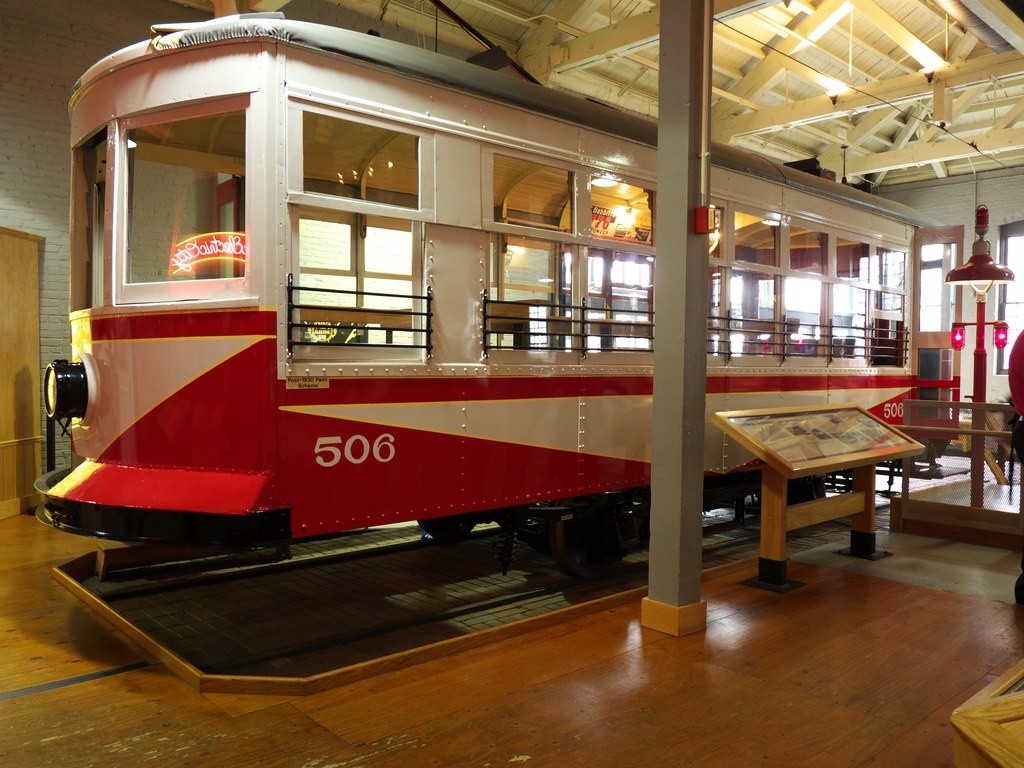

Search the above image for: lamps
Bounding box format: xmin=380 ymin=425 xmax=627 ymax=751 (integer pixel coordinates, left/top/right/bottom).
xmin=945 ymin=204 xmax=1015 ymax=351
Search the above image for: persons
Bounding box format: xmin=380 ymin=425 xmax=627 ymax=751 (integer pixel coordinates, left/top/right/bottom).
xmin=1007 ymin=330 xmax=1024 ymax=603
